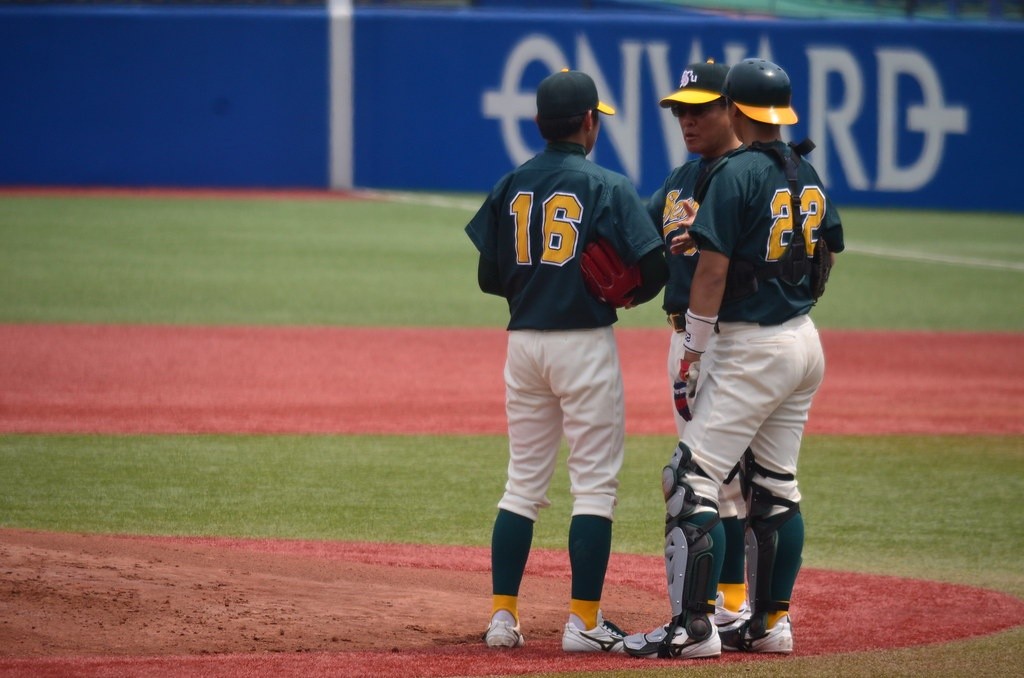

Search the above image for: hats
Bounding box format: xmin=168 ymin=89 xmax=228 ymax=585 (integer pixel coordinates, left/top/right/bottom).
xmin=536 ymin=68 xmax=615 ymax=115
xmin=659 ymin=59 xmax=730 ymax=109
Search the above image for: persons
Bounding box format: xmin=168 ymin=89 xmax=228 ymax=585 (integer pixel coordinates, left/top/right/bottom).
xmin=646 ymin=58 xmax=752 ymax=650
xmin=464 ymin=69 xmax=671 ymax=652
xmin=622 ymin=58 xmax=844 ymax=660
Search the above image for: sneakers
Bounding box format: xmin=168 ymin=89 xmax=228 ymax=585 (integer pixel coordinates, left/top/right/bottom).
xmin=715 ymin=591 xmax=749 ymax=632
xmin=723 ymin=613 xmax=793 ymax=653
xmin=623 ymin=615 xmax=722 ymax=659
xmin=562 ymin=608 xmax=628 ymax=654
xmin=486 ymin=619 xmax=527 ymax=647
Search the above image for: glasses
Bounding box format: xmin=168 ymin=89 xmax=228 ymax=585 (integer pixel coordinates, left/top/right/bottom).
xmin=669 ymin=103 xmax=726 ymax=117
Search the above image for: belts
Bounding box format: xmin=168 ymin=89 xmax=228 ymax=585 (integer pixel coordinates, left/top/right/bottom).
xmin=667 ymin=313 xmax=686 ymax=335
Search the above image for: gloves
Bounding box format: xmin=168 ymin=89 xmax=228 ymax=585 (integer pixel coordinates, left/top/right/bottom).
xmin=673 ymin=359 xmax=700 ymax=422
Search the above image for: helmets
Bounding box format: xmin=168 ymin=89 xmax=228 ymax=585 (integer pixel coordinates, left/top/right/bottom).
xmin=721 ymin=57 xmax=799 ymax=125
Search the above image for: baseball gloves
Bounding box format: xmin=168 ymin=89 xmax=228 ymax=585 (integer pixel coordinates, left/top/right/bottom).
xmin=578 ymin=231 xmax=642 ymax=308
